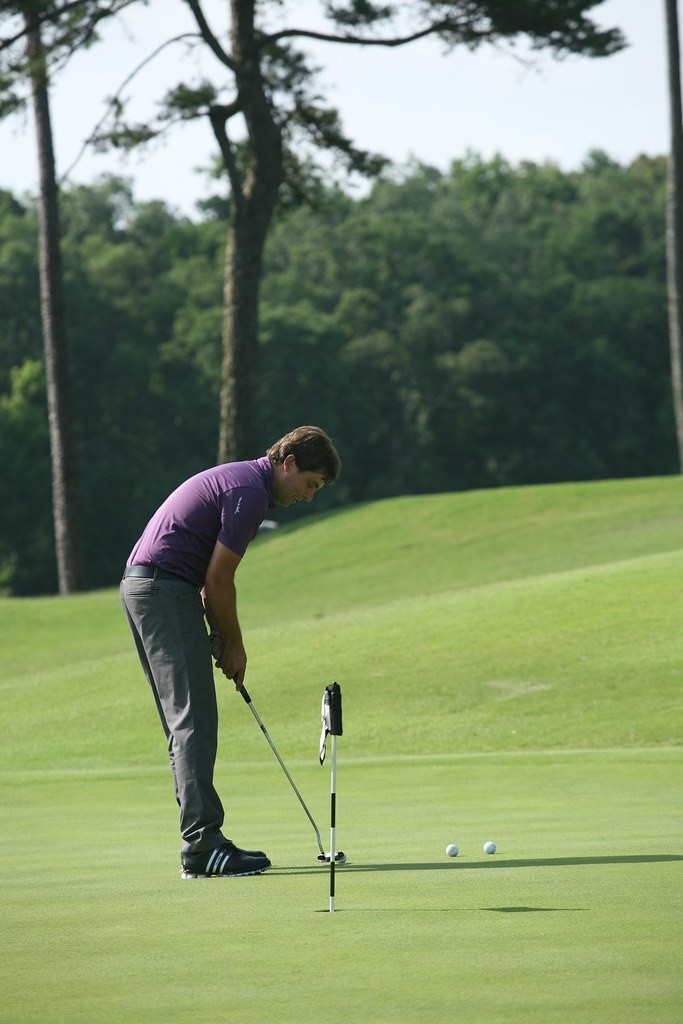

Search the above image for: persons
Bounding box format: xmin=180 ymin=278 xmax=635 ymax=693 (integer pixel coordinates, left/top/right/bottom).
xmin=119 ymin=425 xmax=342 ymax=878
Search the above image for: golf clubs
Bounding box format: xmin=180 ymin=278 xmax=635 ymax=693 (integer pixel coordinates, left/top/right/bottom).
xmin=207 ymin=633 xmax=348 ymax=865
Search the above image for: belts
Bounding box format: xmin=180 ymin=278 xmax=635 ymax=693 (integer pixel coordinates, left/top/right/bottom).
xmin=124 ymin=564 xmax=189 ymax=581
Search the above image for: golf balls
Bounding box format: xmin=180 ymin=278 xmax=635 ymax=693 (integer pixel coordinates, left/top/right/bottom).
xmin=446 ymin=844 xmax=459 ymax=857
xmin=483 ymin=841 xmax=496 ymax=854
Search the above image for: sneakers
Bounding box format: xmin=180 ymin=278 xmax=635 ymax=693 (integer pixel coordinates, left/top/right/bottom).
xmin=225 ymin=839 xmax=270 ymax=857
xmin=181 ymin=842 xmax=272 ymax=879
